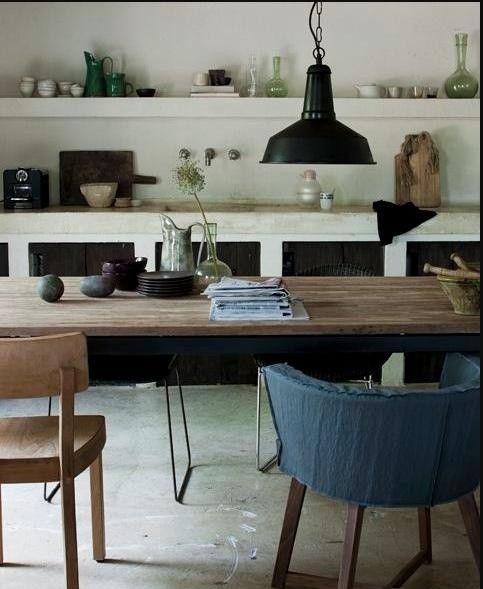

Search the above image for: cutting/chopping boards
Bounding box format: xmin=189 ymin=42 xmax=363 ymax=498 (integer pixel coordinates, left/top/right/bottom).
xmin=59 ymin=151 xmax=156 ymax=207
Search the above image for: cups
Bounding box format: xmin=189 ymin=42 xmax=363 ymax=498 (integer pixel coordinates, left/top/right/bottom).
xmin=319 ymin=193 xmax=334 ymax=210
xmin=408 ymin=86 xmax=424 ymax=98
xmin=193 ymin=72 xmax=208 ymax=86
xmin=425 ymin=87 xmax=439 ymax=98
xmin=387 ymin=87 xmax=403 ymax=98
xmin=208 ymin=70 xmax=227 ymax=86
xmin=105 ymin=72 xmax=133 ymax=97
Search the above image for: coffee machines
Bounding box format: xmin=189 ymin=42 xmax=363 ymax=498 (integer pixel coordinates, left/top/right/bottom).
xmin=3 ymin=167 xmax=50 ymax=210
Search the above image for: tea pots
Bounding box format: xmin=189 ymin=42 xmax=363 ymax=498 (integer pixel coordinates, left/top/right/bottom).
xmin=159 ymin=213 xmax=206 ymax=272
xmin=82 ymin=51 xmax=114 ymax=97
xmin=354 ymin=83 xmax=386 ymax=98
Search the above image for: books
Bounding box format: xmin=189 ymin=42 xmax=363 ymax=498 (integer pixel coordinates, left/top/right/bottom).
xmin=201 ymin=275 xmax=311 ymax=321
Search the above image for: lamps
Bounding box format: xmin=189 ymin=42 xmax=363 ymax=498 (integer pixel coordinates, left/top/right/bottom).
xmin=258 ymin=2 xmax=379 ymax=166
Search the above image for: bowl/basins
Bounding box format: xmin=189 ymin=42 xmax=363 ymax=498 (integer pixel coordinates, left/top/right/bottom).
xmin=79 ymin=182 xmax=118 ymax=207
xmin=102 ymin=256 xmax=148 ymax=292
xmin=15 ymin=76 xmax=85 ymax=98
xmin=115 ymin=198 xmax=144 ymax=208
xmin=136 ymin=89 xmax=155 ymax=97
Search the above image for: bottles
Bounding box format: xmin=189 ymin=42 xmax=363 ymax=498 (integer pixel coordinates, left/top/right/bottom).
xmin=295 ymin=170 xmax=323 ymax=208
xmin=245 ymin=54 xmax=259 ymax=97
xmin=194 ymin=222 xmax=233 ymax=291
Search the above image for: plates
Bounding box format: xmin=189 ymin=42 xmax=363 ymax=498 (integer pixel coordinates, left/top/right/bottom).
xmin=136 ymin=270 xmax=196 ymax=298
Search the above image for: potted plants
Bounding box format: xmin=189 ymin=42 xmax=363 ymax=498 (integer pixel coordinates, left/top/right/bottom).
xmin=174 ymin=158 xmax=234 ymax=290
